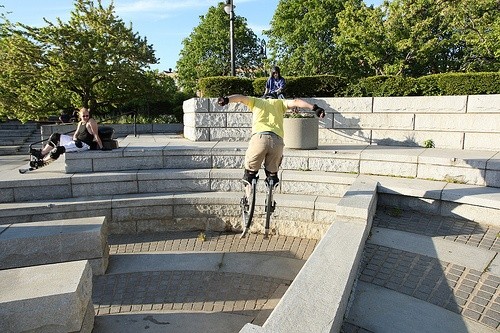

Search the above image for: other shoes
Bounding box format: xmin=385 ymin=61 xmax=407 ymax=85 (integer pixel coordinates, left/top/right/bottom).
xmin=31 ymin=149 xmax=44 ymax=160
xmin=30 ymin=160 xmax=44 ymax=167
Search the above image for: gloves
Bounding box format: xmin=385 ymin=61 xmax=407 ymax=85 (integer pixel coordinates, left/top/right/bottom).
xmin=74 ymin=140 xmax=83 ymax=148
xmin=101 ymin=146 xmax=112 ymax=151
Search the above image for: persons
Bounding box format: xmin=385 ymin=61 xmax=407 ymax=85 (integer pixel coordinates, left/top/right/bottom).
xmin=30 ymin=107 xmax=113 ymax=168
xmin=59 ymin=108 xmax=79 ymax=123
xmin=218 ymin=92 xmax=325 ymax=213
xmin=260 ymin=66 xmax=286 ymax=99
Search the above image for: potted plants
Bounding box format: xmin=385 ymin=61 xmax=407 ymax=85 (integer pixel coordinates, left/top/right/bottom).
xmin=284 ymin=113 xmax=319 ymax=150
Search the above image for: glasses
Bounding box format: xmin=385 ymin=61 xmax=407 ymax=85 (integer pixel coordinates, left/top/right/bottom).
xmin=82 ymin=115 xmax=89 ymax=118
